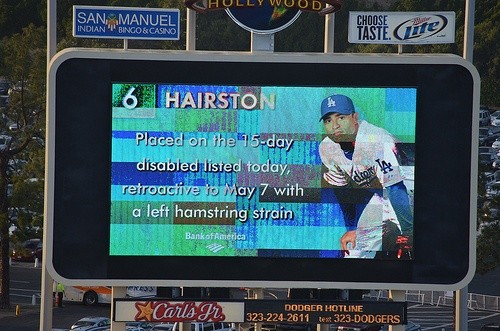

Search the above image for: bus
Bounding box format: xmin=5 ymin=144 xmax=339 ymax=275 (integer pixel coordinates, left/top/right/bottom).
xmin=52 ymin=274 xmax=200 ymax=307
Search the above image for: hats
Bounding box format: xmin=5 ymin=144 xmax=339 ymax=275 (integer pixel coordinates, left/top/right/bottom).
xmin=318 ymin=94 xmax=355 ymax=122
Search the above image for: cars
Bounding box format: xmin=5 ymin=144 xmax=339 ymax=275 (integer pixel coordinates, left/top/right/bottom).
xmin=71 ymin=315 xmax=236 ymax=331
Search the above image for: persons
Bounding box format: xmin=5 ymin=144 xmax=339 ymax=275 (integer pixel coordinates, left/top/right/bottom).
xmin=56 ymin=283 xmax=65 ymax=308
xmin=317 ymin=93 xmax=413 ymax=261
